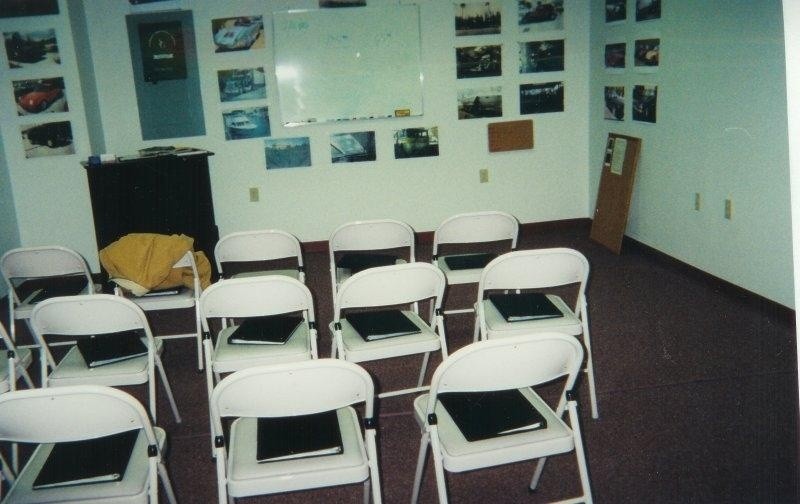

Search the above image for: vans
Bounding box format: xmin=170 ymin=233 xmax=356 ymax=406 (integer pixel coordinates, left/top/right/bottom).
xmin=25 ymin=121 xmax=72 ymax=150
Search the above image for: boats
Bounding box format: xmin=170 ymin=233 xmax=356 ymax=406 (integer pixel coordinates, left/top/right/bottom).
xmin=223 ymin=75 xmax=255 ymax=97
xmin=223 ymin=111 xmax=259 ymax=138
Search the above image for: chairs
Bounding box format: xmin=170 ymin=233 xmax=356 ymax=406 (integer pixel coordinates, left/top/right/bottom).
xmin=1 ymin=209 xmax=599 ymax=503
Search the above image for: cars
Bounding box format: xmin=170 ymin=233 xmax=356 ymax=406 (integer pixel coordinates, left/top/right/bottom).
xmin=13 ymin=78 xmax=66 ymax=114
xmin=601 ymin=1 xmax=662 ymax=124
xmin=458 ymin=0 xmax=566 ymax=122
xmin=330 ymin=134 xmax=371 ymax=163
xmin=212 ymin=18 xmax=262 ymax=51
xmin=3 ymin=36 xmax=48 ymax=65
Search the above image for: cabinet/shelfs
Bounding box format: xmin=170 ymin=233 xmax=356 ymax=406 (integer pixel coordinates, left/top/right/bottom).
xmin=83 ymin=150 xmax=221 ymax=294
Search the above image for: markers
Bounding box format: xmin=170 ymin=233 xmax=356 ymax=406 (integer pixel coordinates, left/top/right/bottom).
xmin=300 ymin=114 xmax=392 ymax=123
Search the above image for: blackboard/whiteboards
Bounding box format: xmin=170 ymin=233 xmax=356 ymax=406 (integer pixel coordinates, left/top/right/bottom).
xmin=272 ymin=3 xmax=424 ymax=128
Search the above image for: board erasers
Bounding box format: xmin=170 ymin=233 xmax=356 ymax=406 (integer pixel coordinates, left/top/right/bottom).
xmin=395 ymin=109 xmax=410 ymax=117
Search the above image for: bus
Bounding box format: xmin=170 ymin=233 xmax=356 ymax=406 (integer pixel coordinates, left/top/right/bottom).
xmin=393 ymin=127 xmax=432 ymax=156
